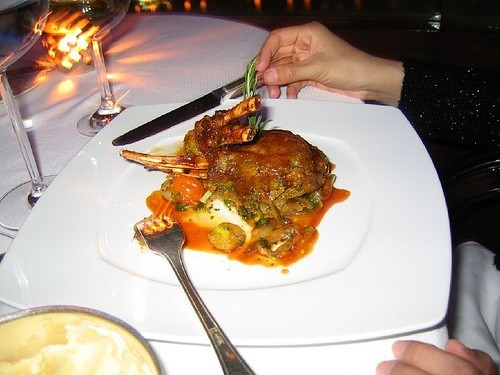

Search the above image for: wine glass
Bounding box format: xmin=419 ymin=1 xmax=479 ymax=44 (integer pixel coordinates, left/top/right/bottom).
xmin=41 ymin=0 xmax=133 ymax=138
xmin=0 ymin=0 xmax=57 ymax=231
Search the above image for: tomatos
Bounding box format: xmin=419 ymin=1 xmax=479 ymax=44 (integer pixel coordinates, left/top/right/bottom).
xmin=170 ymin=175 xmax=205 ymax=201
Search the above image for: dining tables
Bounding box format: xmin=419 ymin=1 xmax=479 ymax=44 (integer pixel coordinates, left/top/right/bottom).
xmin=0 ymin=12 xmax=447 ymax=375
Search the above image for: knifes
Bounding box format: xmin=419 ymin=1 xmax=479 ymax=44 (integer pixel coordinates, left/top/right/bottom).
xmin=111 ymin=57 xmax=294 ymax=147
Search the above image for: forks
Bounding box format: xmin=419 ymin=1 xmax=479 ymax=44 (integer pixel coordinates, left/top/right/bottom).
xmin=136 ymin=213 xmax=256 ymax=375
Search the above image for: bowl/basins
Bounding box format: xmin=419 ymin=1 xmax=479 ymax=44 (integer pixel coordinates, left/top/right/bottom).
xmin=0 ymin=305 xmax=166 ymax=375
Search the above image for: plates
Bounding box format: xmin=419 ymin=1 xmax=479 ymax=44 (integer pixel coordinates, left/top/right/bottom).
xmin=0 ymin=98 xmax=453 ymax=348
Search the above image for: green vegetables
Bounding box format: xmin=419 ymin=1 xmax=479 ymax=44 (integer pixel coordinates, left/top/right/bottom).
xmin=242 ymin=56 xmax=272 ymax=136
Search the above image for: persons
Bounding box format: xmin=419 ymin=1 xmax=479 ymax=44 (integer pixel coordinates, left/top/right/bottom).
xmin=253 ymin=20 xmax=500 ymax=375
